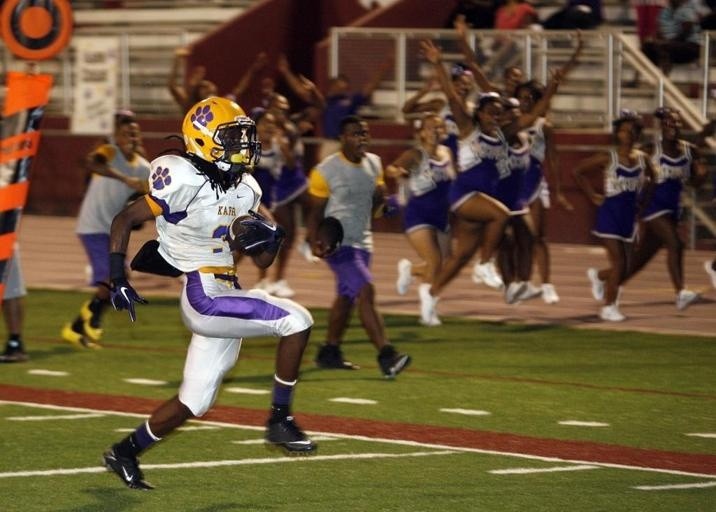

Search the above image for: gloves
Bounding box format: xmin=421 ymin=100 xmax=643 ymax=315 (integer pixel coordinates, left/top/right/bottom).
xmin=239 ymin=209 xmax=279 ymax=253
xmin=108 ymin=278 xmax=146 ymax=323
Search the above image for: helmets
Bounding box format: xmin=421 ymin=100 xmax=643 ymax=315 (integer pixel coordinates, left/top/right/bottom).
xmin=182 ymin=96 xmax=261 ymax=174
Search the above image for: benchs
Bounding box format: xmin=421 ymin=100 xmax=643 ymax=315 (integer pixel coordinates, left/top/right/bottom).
xmin=1 ymin=0 xmax=715 ymax=118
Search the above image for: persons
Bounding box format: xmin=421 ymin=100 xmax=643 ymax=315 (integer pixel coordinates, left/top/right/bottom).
xmin=168 ymin=47 xmax=390 ymax=300
xmin=383 ymin=19 xmax=586 ymax=324
xmin=61 ymin=111 xmax=152 ymax=350
xmin=441 ymin=1 xmax=606 ymax=76
xmin=1 ymin=40 xmax=32 ymax=363
xmin=626 ymin=1 xmax=715 ymax=100
xmin=101 ymin=96 xmax=317 ymax=490
xmin=304 ymin=117 xmax=410 ymax=379
xmin=573 ymin=107 xmax=715 ymax=321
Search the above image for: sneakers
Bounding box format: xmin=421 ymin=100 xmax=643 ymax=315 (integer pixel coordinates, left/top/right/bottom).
xmin=706 ymin=262 xmax=716 ymax=288
xmin=419 ymin=283 xmax=440 ymax=327
xmin=380 ymin=354 xmax=410 ymax=377
xmin=315 ymin=348 xmax=359 ymax=370
xmin=62 ymin=300 xmax=104 ymax=349
xmin=298 ymin=243 xmax=322 ymax=263
xmin=255 ymin=278 xmax=295 ymax=297
xmin=676 ymin=291 xmax=698 ymax=310
xmin=472 ymin=260 xmax=559 ymax=304
xmin=265 ymin=420 xmax=316 ymax=456
xmin=100 ymin=443 xmax=156 ymax=490
xmin=600 ymin=286 xmax=625 ymax=321
xmin=1 ymin=336 xmax=25 ymax=362
xmin=397 ymin=259 xmax=417 ymax=294
xmin=588 ymin=267 xmax=605 ymax=300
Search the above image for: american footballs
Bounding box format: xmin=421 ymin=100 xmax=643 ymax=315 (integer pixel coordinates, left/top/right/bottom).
xmin=230 ymin=216 xmax=266 ymax=256
xmin=317 ymin=216 xmax=343 ymax=250
